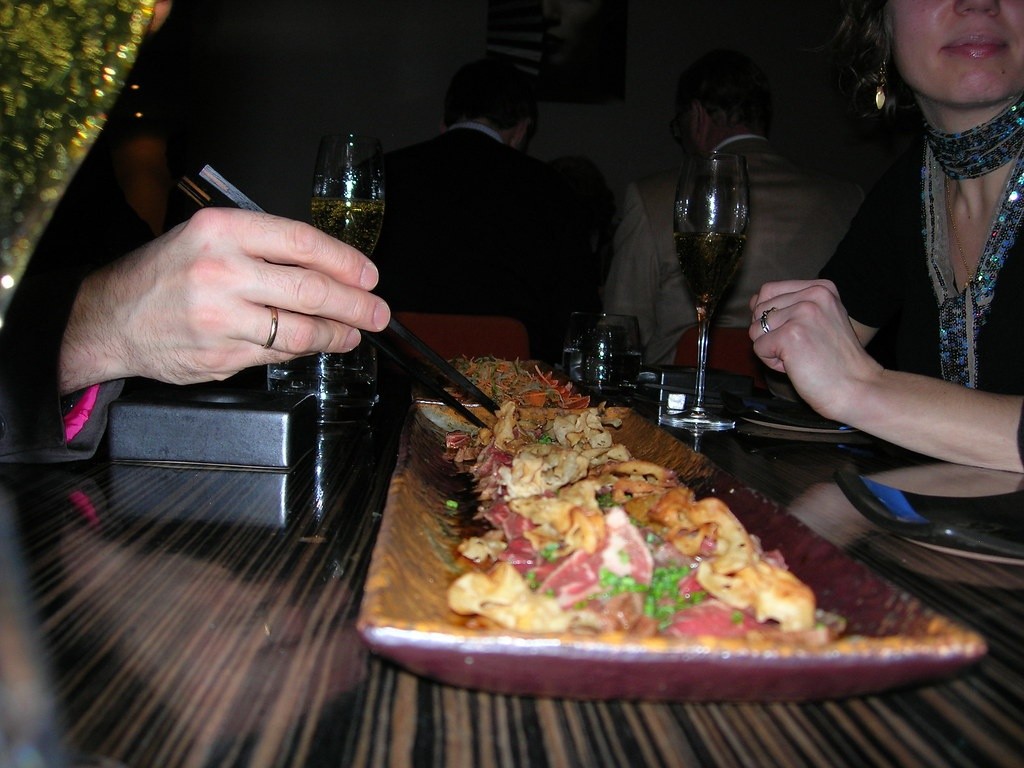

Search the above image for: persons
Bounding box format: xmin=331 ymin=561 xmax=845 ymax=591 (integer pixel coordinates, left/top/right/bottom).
xmin=749 ymin=0 xmax=1024 ymax=473
xmin=782 ymin=463 xmax=1024 ymax=561
xmin=0 ymin=465 xmax=370 ymax=736
xmin=0 ymin=0 xmax=392 ymax=463
xmin=58 ymin=109 xmax=188 ymax=268
xmin=339 ymin=48 xmax=865 ymax=367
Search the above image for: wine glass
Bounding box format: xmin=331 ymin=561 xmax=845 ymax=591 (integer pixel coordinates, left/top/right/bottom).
xmin=658 ymin=150 xmax=752 ymax=431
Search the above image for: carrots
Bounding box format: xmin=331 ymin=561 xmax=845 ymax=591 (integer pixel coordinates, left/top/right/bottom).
xmin=453 ymin=353 xmax=584 ymax=409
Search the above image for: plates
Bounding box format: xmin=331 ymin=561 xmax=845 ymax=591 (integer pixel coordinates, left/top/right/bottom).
xmin=831 ymin=469 xmax=1024 ymax=565
xmin=357 ymin=406 xmax=988 ymax=698
xmin=742 ymin=413 xmax=860 ymax=435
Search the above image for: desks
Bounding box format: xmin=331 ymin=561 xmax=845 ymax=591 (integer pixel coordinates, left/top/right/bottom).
xmin=0 ymin=376 xmax=1024 ymax=767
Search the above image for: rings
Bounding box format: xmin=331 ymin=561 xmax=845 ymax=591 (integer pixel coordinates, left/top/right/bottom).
xmin=760 ymin=306 xmax=777 ymax=334
xmin=261 ymin=306 xmax=278 ymax=350
xmin=261 ymin=619 xmax=284 ymax=652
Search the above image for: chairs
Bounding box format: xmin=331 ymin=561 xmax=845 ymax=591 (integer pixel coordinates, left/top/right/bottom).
xmin=394 ymin=313 xmax=531 ymax=359
xmin=676 ymin=328 xmax=765 ymax=385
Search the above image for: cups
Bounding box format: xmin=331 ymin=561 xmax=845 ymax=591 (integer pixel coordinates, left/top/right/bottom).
xmin=0 ymin=0 xmax=151 ymax=325
xmin=565 ymin=310 xmax=642 ymax=398
xmin=307 ymin=131 xmax=386 ymax=263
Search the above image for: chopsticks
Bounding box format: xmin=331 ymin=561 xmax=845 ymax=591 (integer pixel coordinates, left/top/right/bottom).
xmin=175 ymin=161 xmax=500 ymax=429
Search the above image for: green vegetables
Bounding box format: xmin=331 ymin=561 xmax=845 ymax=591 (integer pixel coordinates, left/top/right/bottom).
xmin=444 ymin=433 xmax=746 ymax=630
